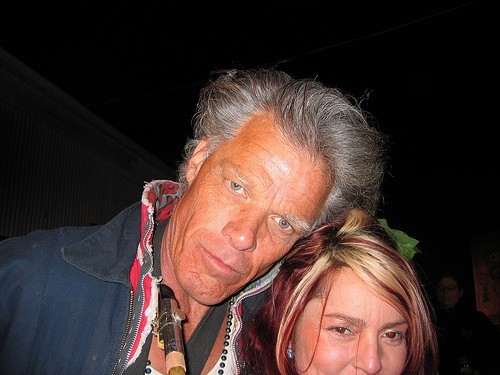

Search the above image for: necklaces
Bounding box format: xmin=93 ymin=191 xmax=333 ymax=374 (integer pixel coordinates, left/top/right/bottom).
xmin=145 ymin=296 xmax=235 ymax=375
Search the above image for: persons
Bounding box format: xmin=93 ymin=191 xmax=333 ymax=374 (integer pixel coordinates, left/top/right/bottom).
xmin=430 ymin=272 xmax=499 ymax=375
xmin=0 ymin=67 xmax=389 ymax=375
xmin=240 ymin=204 xmax=439 ymax=375
